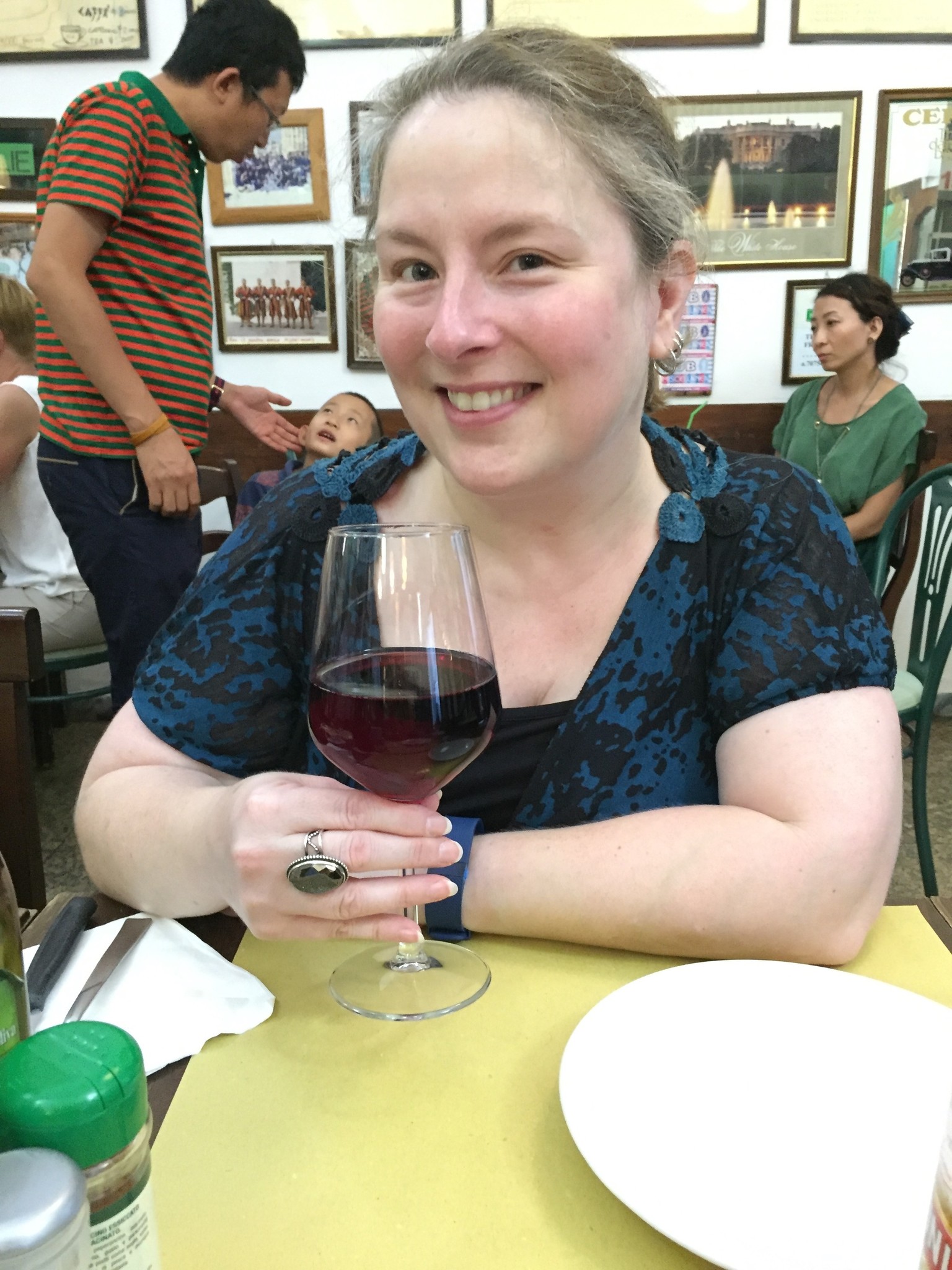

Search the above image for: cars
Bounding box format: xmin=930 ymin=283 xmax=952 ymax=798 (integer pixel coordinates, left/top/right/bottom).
xmin=899 ymin=247 xmax=952 ymax=287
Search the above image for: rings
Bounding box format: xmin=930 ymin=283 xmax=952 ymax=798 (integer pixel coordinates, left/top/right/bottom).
xmin=285 ymin=830 xmax=349 ymax=896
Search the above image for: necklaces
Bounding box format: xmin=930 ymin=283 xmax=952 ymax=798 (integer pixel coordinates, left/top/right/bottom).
xmin=815 ymin=370 xmax=883 ymax=482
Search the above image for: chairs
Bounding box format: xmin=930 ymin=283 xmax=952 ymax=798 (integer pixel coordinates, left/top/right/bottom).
xmin=871 ymin=463 xmax=952 ymax=897
xmin=0 ymin=459 xmax=242 ymax=913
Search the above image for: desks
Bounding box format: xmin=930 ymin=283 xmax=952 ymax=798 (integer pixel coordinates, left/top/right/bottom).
xmin=18 ymin=893 xmax=952 ymax=1270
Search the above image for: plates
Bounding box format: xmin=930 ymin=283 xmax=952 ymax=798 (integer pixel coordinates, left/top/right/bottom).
xmin=557 ymin=958 xmax=952 ymax=1270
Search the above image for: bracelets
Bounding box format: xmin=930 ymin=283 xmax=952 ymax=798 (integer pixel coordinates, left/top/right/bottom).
xmin=208 ymin=376 xmax=225 ymax=413
xmin=129 ymin=421 xmax=172 ymax=440
xmin=425 ymin=817 xmax=486 ymax=941
xmin=132 ymin=414 xmax=168 ymax=447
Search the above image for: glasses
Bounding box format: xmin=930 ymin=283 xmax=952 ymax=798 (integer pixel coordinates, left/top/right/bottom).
xmin=231 ymin=65 xmax=282 ymax=132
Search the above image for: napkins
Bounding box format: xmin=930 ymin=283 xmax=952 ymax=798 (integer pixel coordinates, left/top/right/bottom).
xmin=22 ymin=913 xmax=276 ymax=1075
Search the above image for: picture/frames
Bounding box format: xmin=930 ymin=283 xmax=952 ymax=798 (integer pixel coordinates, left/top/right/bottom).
xmin=486 ymin=0 xmax=766 ymax=48
xmin=780 ymin=279 xmax=835 ymax=384
xmin=185 ymin=0 xmax=463 ymax=50
xmin=790 ymin=0 xmax=952 ymax=44
xmin=0 ymin=0 xmax=149 ymax=62
xmin=867 ymin=88 xmax=952 ymax=304
xmin=658 ymin=91 xmax=862 ymax=268
xmin=205 ymin=108 xmax=331 ymax=226
xmin=344 ymin=240 xmax=385 ymax=370
xmin=210 ymin=244 xmax=339 ymax=353
xmin=349 ymin=100 xmax=386 ymax=215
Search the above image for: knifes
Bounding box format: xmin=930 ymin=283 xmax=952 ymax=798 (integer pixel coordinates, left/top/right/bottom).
xmin=25 ymin=896 xmax=97 ymax=1033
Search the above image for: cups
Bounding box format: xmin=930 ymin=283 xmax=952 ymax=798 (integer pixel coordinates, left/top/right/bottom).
xmin=920 ymin=1134 xmax=952 ymax=1270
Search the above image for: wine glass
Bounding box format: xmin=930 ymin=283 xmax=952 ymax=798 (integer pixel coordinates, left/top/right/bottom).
xmin=306 ymin=523 xmax=502 ymax=1021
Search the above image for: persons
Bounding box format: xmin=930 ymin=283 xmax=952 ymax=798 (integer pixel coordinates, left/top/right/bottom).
xmin=230 ymin=149 xmax=318 ymax=330
xmin=72 ymin=20 xmax=907 ymax=972
xmin=0 ymin=274 xmax=927 ymax=965
xmin=33 ymin=0 xmax=311 ymax=921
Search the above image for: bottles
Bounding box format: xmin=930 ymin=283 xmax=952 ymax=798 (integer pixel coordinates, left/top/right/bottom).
xmin=0 ymin=850 xmax=31 ymax=1055
xmin=0 ymin=1021 xmax=159 ymax=1270
xmin=0 ymin=1147 xmax=91 ymax=1270
